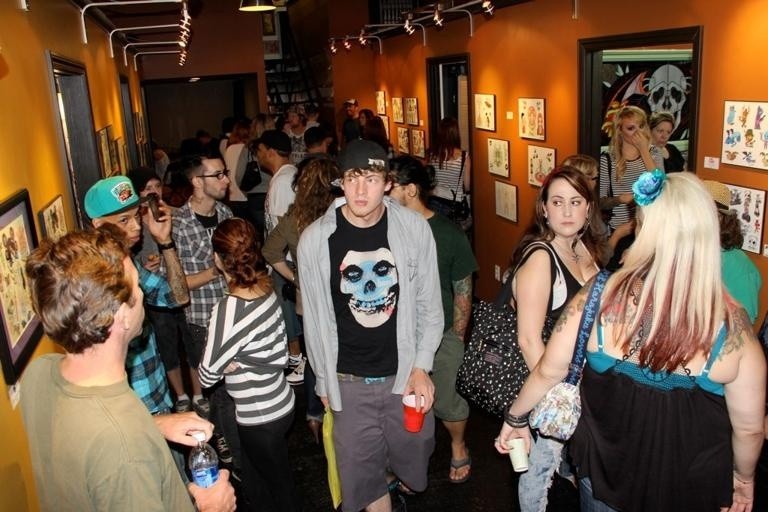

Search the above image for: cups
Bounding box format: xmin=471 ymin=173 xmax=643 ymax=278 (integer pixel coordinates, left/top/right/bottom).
xmin=193 ymin=169 xmax=231 ymax=180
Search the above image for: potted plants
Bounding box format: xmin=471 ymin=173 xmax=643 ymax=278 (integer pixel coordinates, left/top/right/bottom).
xmin=95 ymin=110 xmax=152 ymax=181
xmin=600 ymin=48 xmax=692 ymax=160
xmin=0 ymin=187 xmax=52 ymax=386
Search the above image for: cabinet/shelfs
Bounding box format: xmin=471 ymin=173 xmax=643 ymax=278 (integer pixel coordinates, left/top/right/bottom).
xmin=187 ymin=430 xmax=220 ymax=489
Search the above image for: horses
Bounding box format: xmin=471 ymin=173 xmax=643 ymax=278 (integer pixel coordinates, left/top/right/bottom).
xmin=148 ymin=191 xmax=166 ymax=222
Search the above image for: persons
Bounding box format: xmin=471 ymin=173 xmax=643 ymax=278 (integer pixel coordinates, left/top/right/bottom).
xmin=561 ymin=154 xmax=636 ymax=270
xmin=701 ymin=179 xmax=763 ymax=327
xmin=500 ymin=167 xmax=600 ymax=512
xmin=18 ymin=98 xmax=480 ymax=511
xmin=597 ymin=105 xmax=665 ymax=265
xmin=494 ymin=170 xmax=768 ymax=512
xmin=649 ymin=111 xmax=685 ymax=171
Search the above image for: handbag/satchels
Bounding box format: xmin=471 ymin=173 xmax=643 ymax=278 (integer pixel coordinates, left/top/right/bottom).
xmin=502 ymin=403 xmax=532 ymax=429
xmin=735 ymin=476 xmax=754 ymax=484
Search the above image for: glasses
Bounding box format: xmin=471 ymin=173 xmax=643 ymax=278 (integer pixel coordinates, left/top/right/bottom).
xmin=78 ymin=1 xmax=192 ymax=73
xmin=237 ymin=1 xmax=276 ymax=13
xmin=328 ymin=0 xmax=497 ymax=57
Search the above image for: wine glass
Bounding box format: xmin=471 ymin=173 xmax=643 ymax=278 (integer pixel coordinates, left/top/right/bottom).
xmin=494 ymin=437 xmax=499 ymax=443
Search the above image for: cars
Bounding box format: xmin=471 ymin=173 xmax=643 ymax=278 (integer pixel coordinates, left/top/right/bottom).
xmin=507 ymin=437 xmax=529 ymax=473
xmin=402 ymin=393 xmax=427 ymax=433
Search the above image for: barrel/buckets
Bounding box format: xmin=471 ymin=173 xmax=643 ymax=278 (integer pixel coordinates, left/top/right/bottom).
xmin=447 ymin=448 xmax=475 ymax=485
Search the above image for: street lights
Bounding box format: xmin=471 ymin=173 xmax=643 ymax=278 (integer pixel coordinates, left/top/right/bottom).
xmin=455 ymin=299 xmax=555 ymax=419
xmin=449 ymin=197 xmax=475 ymax=229
xmin=528 ymin=380 xmax=582 ymax=442
xmin=599 ymin=195 xmax=615 ymax=225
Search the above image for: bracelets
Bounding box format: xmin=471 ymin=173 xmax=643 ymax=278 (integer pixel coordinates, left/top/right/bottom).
xmin=156 ymin=239 xmax=175 ymax=250
xmin=502 ymin=403 xmax=532 ymax=429
xmin=735 ymin=476 xmax=754 ymax=484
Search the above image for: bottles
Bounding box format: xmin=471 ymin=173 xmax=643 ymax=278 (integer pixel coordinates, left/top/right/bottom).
xmin=344 ymin=97 xmax=359 ymax=106
xmin=251 ymin=129 xmax=291 ymax=147
xmin=128 ymin=166 xmax=163 ymax=193
xmin=151 ymin=148 xmax=168 ymax=162
xmin=339 ymin=139 xmax=391 ymax=175
xmin=84 ymin=176 xmax=151 ymax=218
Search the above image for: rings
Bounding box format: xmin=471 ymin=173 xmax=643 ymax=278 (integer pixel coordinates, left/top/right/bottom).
xmin=494 ymin=437 xmax=499 ymax=443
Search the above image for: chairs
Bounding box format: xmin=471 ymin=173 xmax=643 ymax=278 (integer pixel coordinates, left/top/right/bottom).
xmin=285 ymin=353 xmax=303 ymax=369
xmin=214 ymin=435 xmax=235 ymax=464
xmin=192 ymin=396 xmax=212 ymax=419
xmin=286 ymin=356 xmax=308 ymax=385
xmin=175 ymin=398 xmax=191 ymax=413
